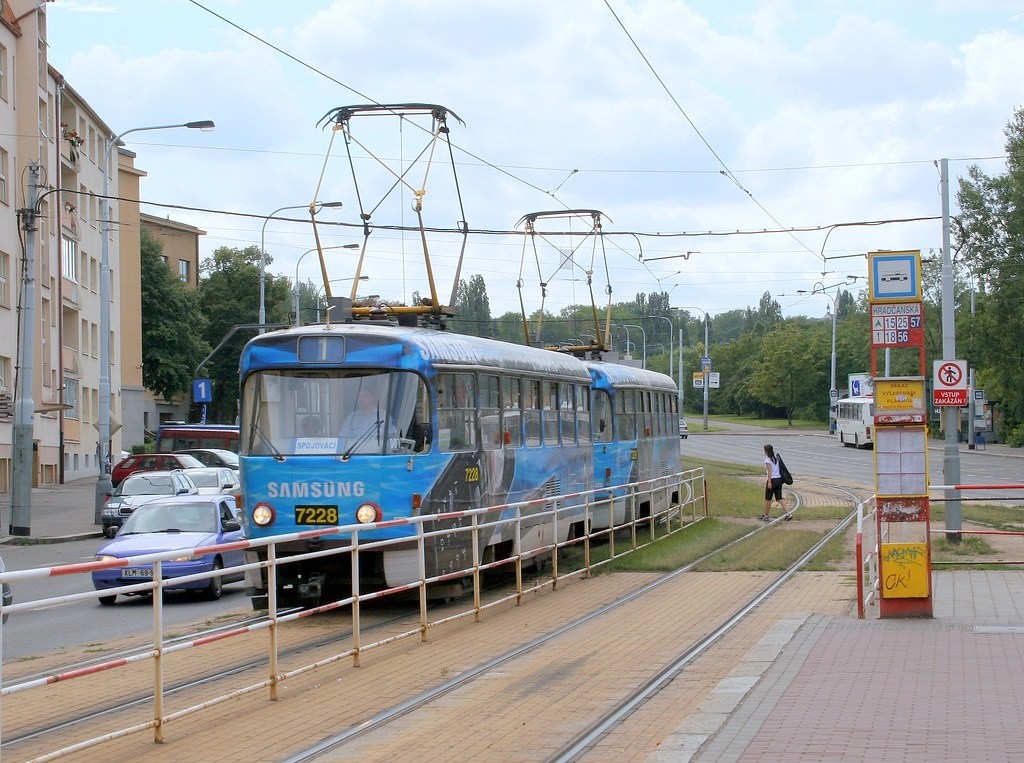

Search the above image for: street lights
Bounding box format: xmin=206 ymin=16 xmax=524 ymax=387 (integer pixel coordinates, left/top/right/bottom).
xmin=93 ymin=119 xmax=218 ymax=525
xmin=670 ymin=306 xmax=710 ymax=430
xmin=846 ymin=275 xmax=891 ymax=379
xmin=559 ymin=323 xmax=664 ymax=371
xmin=640 ymin=315 xmax=673 ymax=381
xmin=294 ymin=243 xmax=359 ymax=327
xmin=922 ymin=259 xmax=976 ymax=450
xmin=258 ymin=201 xmax=343 ymax=335
xmin=797 ymin=290 xmax=838 ymax=390
xmin=316 ymin=276 xmax=370 ymax=326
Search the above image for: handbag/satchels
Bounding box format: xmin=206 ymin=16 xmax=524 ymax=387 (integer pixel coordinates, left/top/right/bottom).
xmin=776 ymin=453 xmax=793 ymax=485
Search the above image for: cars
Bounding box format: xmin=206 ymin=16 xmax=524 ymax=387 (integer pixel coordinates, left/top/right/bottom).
xmin=99 ymin=427 xmax=242 ymax=536
xmin=679 ymin=419 xmax=688 ymax=439
xmin=91 ymin=494 xmax=247 ymax=606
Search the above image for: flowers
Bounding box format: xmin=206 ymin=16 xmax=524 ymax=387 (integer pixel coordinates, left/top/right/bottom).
xmin=65 ymin=201 xmax=76 ymax=212
xmin=61 ymin=120 xmax=85 ymax=146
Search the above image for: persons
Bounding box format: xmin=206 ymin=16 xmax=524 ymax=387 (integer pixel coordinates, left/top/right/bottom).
xmin=758 ymin=444 xmax=793 ymax=521
xmin=339 ymin=383 xmax=399 ymax=448
xmin=302 ymin=416 xmax=320 ymax=436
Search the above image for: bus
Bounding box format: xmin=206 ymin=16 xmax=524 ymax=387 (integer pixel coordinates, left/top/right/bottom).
xmin=240 ymin=101 xmax=596 ymax=611
xmin=833 ymin=396 xmax=875 ymax=449
xmin=515 ymin=209 xmax=684 ymax=542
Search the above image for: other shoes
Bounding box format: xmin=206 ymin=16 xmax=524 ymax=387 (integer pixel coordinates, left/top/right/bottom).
xmin=758 ymin=514 xmax=769 ymax=521
xmin=784 ymin=513 xmax=793 ymax=520
